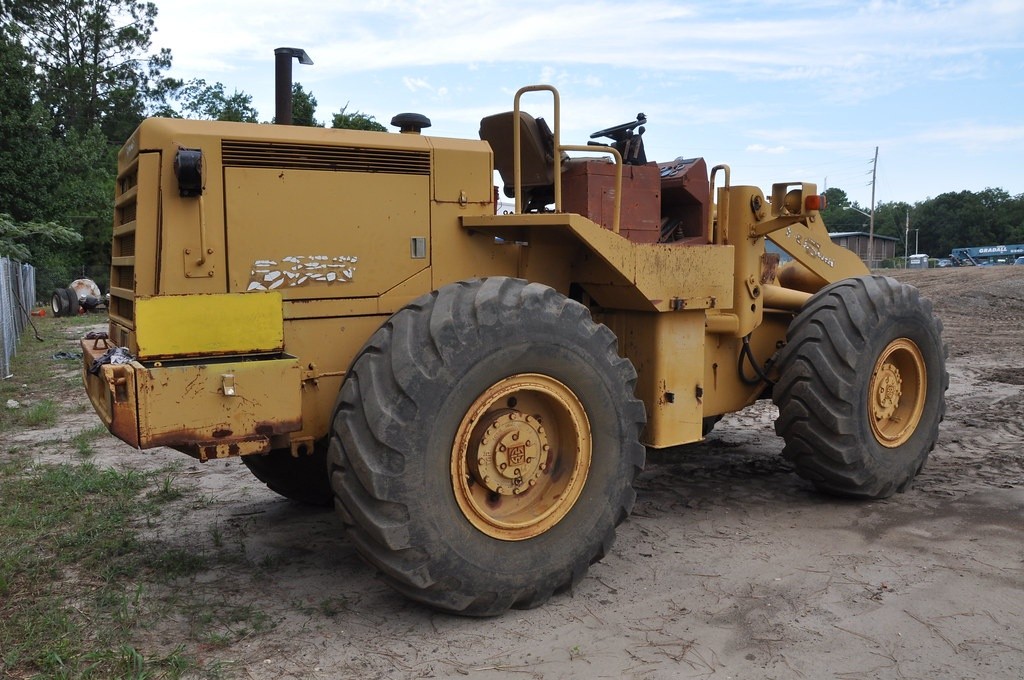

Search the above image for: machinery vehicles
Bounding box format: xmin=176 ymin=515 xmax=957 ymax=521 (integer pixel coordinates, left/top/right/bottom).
xmin=80 ymin=50 xmax=950 ymax=618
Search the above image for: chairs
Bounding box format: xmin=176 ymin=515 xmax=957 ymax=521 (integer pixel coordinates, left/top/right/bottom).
xmin=478 ymin=111 xmax=574 ymax=210
